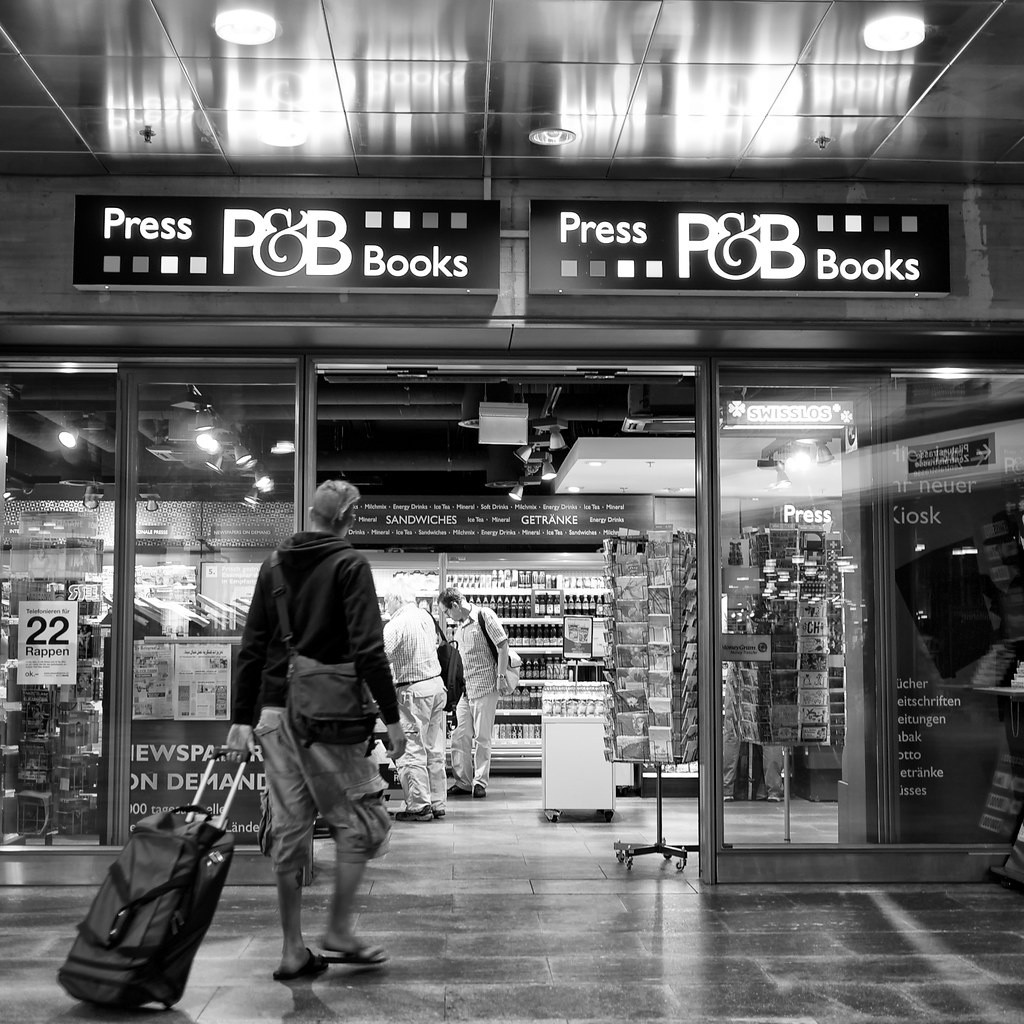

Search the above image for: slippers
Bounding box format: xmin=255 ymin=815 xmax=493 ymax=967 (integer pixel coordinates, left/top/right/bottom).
xmin=318 ymin=936 xmax=387 ymax=965
xmin=272 ymin=944 xmax=327 ymax=980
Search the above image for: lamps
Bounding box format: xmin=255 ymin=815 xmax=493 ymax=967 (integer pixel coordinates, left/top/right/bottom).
xmin=774 ymin=462 xmax=791 ymax=489
xmin=171 ymin=394 xmax=275 ymax=504
xmin=532 ymin=417 xmax=568 ymax=450
xmin=58 ymin=419 xmax=89 ymax=447
xmin=508 ymin=477 xmax=541 ymax=501
xmin=139 ymin=488 xmax=161 ymax=512
xmin=815 ymin=441 xmax=834 ymax=464
xmin=83 ymin=486 xmax=105 ymax=509
xmin=514 ymin=435 xmax=550 ymax=463
xmin=528 ymin=452 xmax=557 ymax=480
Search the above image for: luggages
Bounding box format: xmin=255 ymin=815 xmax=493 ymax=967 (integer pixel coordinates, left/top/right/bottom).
xmin=58 ymin=749 xmax=252 ymax=1006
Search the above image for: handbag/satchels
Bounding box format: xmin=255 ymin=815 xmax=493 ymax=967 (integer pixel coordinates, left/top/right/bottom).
xmin=479 ymin=611 xmax=522 ymax=696
xmin=286 ymin=655 xmax=381 ymax=746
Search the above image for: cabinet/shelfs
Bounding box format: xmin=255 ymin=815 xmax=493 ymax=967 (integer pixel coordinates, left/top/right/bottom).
xmin=369 ymin=553 xmax=605 ymax=772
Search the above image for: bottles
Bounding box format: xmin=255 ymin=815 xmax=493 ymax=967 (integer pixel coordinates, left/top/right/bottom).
xmin=519 ymin=657 xmax=574 ymax=679
xmin=492 ymin=723 xmax=541 ymax=739
xmin=534 ymin=595 xmax=561 ymax=616
xmin=497 ymin=686 xmax=542 ymax=708
xmin=467 ymin=595 xmax=531 ymax=618
xmin=504 ymin=624 xmax=563 ymax=646
xmin=563 ymin=592 xmax=614 ymax=616
xmin=446 ymin=569 xmax=605 ymax=589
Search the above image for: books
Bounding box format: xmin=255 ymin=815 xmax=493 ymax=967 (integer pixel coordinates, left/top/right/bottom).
xmin=722 ymin=523 xmax=846 ymax=745
xmin=0 ymin=538 xmax=252 ymax=835
xmin=379 ymin=524 xmax=698 ymax=773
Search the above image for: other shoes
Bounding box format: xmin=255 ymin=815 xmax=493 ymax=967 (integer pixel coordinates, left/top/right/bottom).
xmin=447 ymin=785 xmax=472 ymax=795
xmin=473 ymin=783 xmax=486 ymax=798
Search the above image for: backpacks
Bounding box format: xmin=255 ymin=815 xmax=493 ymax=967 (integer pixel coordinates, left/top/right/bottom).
xmin=426 ymin=609 xmax=464 ymax=712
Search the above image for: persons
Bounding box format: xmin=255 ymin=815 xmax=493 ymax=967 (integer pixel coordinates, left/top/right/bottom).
xmin=228 ymin=480 xmax=408 ymax=978
xmin=382 ymin=577 xmax=447 ymax=822
xmin=722 ymin=663 xmax=785 ymax=802
xmin=437 ymin=587 xmax=509 ymax=795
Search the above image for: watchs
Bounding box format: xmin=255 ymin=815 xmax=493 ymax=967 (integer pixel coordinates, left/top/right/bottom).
xmin=496 ymin=673 xmax=506 ymax=679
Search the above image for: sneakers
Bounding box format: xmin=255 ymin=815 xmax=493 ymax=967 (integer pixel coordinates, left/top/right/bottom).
xmin=432 ymin=809 xmax=445 ymax=817
xmin=396 ymin=805 xmax=434 ymax=821
